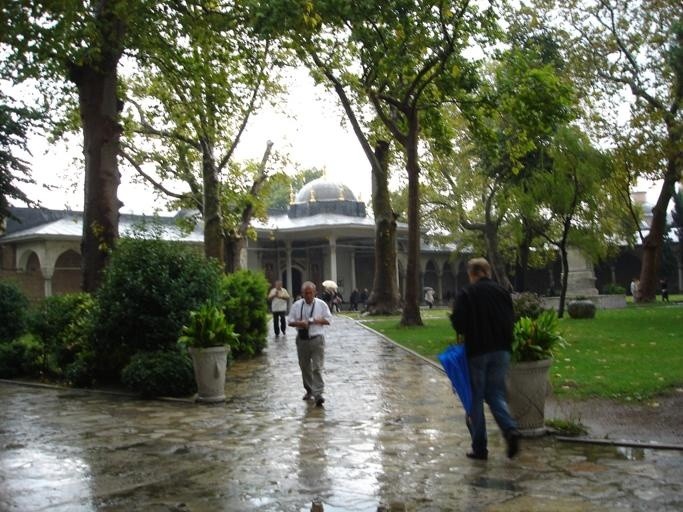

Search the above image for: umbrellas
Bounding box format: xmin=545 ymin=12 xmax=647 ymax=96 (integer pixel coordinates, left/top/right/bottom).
xmin=322 ymin=280 xmax=338 ymax=289
xmin=438 ymin=332 xmax=473 ymax=418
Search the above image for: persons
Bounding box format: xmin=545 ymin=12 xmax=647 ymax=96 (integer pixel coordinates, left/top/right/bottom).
xmin=659 ymin=280 xmax=669 ymax=303
xmin=268 ymin=280 xmax=289 ymax=336
xmin=320 ymin=288 xmax=345 ymax=313
xmin=424 ymin=290 xmax=436 ymax=308
xmin=350 ymin=289 xmax=360 ymax=312
xmin=361 ymin=288 xmax=370 ymax=304
xmin=287 ymin=280 xmax=331 ymax=406
xmin=449 ymin=257 xmax=522 ymax=459
xmin=630 ymin=278 xmax=640 ymax=302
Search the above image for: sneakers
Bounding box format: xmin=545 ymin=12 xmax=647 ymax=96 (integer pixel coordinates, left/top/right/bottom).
xmin=302 ymin=393 xmax=324 ymax=408
xmin=275 ymin=331 xmax=286 ymax=337
xmin=506 ymin=430 xmax=521 ymax=460
xmin=466 ymin=447 xmax=489 ymax=460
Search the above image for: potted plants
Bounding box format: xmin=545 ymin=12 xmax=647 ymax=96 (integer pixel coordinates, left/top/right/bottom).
xmin=503 ymin=305 xmax=573 ymax=442
xmin=174 ymin=303 xmax=242 ymax=405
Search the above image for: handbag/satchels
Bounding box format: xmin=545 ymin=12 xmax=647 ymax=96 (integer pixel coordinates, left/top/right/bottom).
xmin=298 ymin=328 xmax=310 ymax=340
